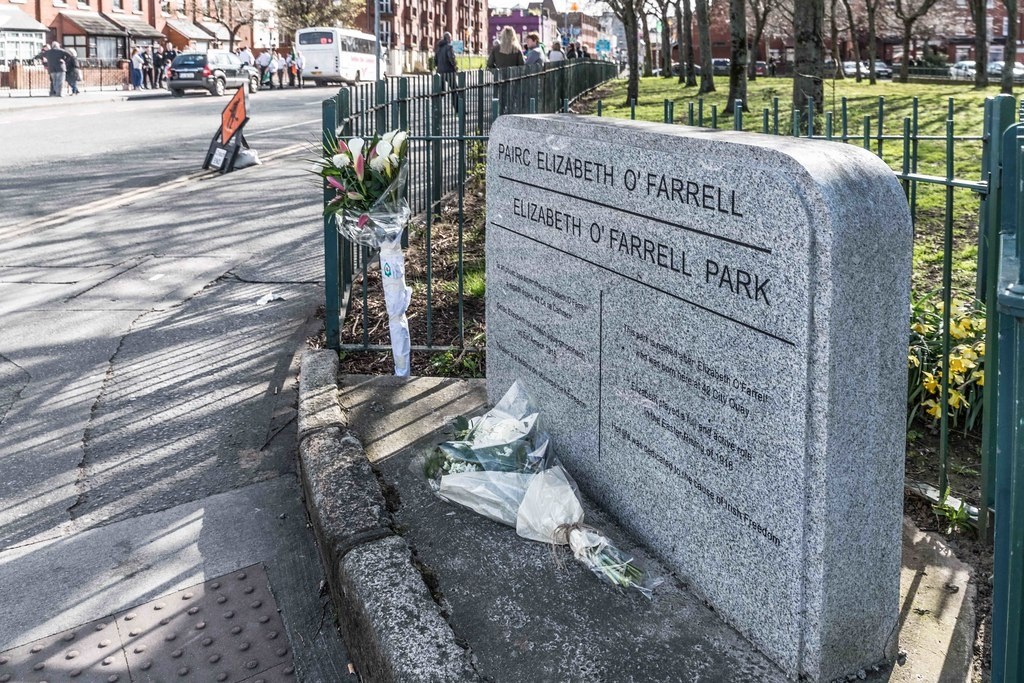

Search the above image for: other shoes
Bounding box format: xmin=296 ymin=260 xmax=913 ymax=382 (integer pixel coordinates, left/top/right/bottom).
xmin=76 ymin=89 xmax=81 ymax=95
xmin=159 ymin=84 xmax=163 ymax=88
xmin=288 ymin=83 xmax=294 ymax=86
xmin=48 ymin=91 xmax=64 ymax=98
xmin=296 ymin=85 xmax=302 ymax=89
xmin=456 ymin=113 xmax=469 ymax=117
xmin=277 ymin=84 xmax=283 ymax=89
xmin=69 ymin=93 xmax=76 ymax=96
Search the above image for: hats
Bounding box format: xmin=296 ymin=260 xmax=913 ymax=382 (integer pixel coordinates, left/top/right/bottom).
xmin=272 ymin=44 xmax=276 ymax=46
xmin=272 ymin=48 xmax=276 ymax=51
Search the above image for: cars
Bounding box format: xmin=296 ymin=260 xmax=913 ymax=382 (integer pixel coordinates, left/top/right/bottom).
xmin=986 ymin=60 xmax=1024 ymax=81
xmin=841 ymin=61 xmax=871 ymax=79
xmin=653 ymin=61 xmax=702 ymax=76
xmin=865 ymin=62 xmax=893 ymax=78
xmin=949 ymin=60 xmax=978 ymax=82
xmin=755 ymin=61 xmax=767 ymax=77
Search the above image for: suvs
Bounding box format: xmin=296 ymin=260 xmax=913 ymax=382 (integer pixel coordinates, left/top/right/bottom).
xmin=167 ymin=49 xmax=260 ymax=97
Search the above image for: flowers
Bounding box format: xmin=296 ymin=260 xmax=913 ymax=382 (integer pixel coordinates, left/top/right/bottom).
xmin=297 ymin=125 xmax=422 ymax=379
xmin=409 ymin=376 xmax=664 ymax=597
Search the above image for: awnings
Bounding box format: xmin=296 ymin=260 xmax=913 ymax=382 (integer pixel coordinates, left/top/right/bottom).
xmin=60 ymin=11 xmax=129 ymax=37
xmin=198 ymin=21 xmax=242 ymax=42
xmin=164 ymin=18 xmax=215 ymax=41
xmin=103 ymin=12 xmax=167 ymax=39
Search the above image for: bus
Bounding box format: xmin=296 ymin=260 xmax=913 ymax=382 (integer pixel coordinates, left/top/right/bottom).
xmin=295 ymin=27 xmax=387 ymax=87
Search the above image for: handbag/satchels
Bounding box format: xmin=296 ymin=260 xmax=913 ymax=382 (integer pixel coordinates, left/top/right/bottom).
xmin=262 ymin=70 xmax=271 ymax=83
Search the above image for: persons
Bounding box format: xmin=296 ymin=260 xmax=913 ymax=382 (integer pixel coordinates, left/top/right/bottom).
xmin=237 ymin=42 xmax=304 ymax=90
xmin=33 ymin=41 xmax=81 ymax=98
xmin=434 ymin=31 xmax=464 ymax=114
xmin=128 ymin=42 xmax=178 ymax=91
xmin=566 ymin=40 xmax=591 ymax=59
xmin=548 ymin=41 xmax=568 ymax=61
xmin=487 ymin=25 xmax=526 ymax=116
xmin=520 ymin=33 xmax=546 ymax=114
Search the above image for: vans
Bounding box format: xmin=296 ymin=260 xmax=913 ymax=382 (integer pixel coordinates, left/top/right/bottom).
xmin=711 ymin=58 xmax=731 ymax=76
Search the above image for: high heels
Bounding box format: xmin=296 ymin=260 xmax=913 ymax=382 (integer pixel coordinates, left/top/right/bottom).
xmin=144 ymin=84 xmax=149 ymax=89
xmin=152 ymin=85 xmax=158 ymax=89
xmin=154 ymin=83 xmax=157 ymax=86
